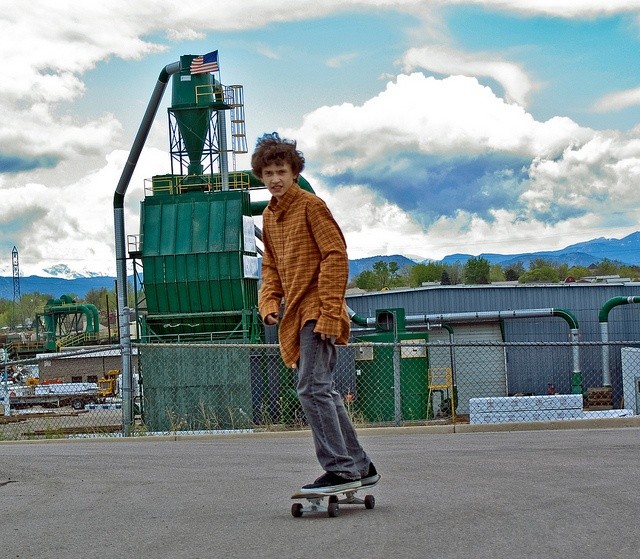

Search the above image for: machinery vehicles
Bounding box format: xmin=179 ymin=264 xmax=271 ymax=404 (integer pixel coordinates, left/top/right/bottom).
xmin=99 ymin=369 xmax=121 ymax=396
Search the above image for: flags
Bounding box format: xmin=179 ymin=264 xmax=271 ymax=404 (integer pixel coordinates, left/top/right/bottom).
xmin=190 ymin=50 xmax=219 ymax=74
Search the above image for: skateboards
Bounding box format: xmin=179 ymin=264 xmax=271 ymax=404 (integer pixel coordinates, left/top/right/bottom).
xmin=290 ymin=473 xmax=382 ymax=518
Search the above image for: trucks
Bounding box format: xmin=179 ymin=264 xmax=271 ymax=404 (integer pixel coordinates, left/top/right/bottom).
xmin=0 ymin=381 xmax=98 ymax=409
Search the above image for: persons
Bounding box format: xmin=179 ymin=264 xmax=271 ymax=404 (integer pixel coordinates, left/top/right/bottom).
xmin=251 ymin=131 xmax=381 ymax=494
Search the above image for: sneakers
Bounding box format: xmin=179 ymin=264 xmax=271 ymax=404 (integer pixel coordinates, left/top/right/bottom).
xmin=300 ymin=472 xmax=362 ymax=493
xmin=361 ymin=463 xmax=379 ymax=485
